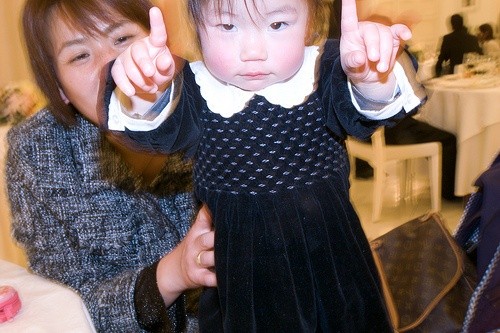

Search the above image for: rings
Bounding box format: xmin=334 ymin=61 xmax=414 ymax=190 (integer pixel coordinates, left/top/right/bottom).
xmin=197 ymin=250 xmax=204 ymax=267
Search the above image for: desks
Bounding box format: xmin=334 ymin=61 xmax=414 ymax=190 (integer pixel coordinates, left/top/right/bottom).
xmin=412 ymin=74 xmax=500 ymax=209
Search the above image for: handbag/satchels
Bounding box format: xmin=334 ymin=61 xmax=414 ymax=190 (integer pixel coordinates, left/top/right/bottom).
xmin=368 ymin=212 xmax=481 ymax=333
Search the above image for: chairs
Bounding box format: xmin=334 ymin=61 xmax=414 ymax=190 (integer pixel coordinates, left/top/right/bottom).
xmin=343 ymin=125 xmax=443 ymax=223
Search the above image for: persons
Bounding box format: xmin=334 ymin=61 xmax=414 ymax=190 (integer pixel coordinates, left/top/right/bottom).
xmin=478 ymin=24 xmax=500 ymax=57
xmin=435 ymin=14 xmax=482 ymax=76
xmin=384 ymin=50 xmax=466 ymax=204
xmin=102 ymin=0 xmax=428 ymax=333
xmin=4 ymin=0 xmax=216 ymax=333
